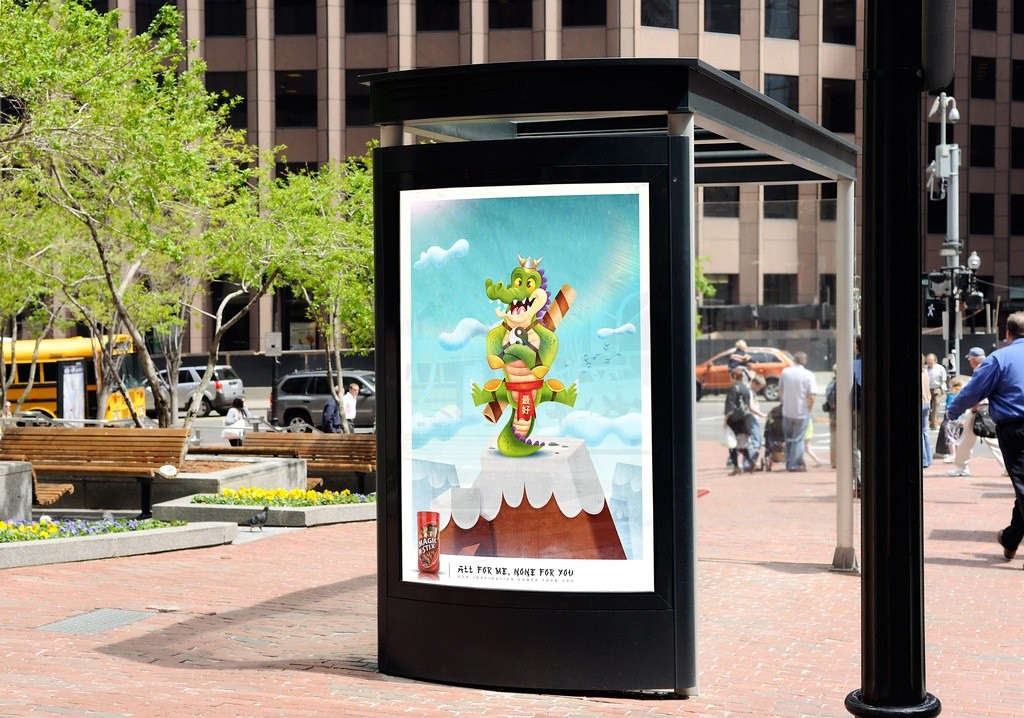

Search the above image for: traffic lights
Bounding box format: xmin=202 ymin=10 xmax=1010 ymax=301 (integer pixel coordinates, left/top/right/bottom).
xmin=926 ymin=300 xmax=942 ymax=328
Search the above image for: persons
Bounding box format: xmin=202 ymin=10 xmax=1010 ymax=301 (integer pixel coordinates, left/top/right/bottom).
xmin=826 ymin=364 xmax=837 ymax=468
xmin=724 ymin=340 xmax=759 ymax=384
xmin=225 ymin=397 xmax=250 ymax=446
xmin=946 ymin=313 xmax=1024 ymax=570
xmin=742 ymin=373 xmax=767 ymax=473
xmin=922 ymin=355 xmax=932 ymax=468
xmin=946 ymin=347 xmax=1009 ymax=476
xmin=779 ymin=351 xmax=822 ymax=472
xmin=944 ymin=378 xmax=962 ymax=462
xmin=853 ymin=334 xmax=862 ymax=497
xmin=723 ymin=369 xmax=756 ymax=475
xmin=343 ymin=383 xmax=359 ymax=433
xmin=322 ymin=386 xmax=344 ymax=433
xmin=924 ymin=353 xmax=946 ymax=431
xmin=0 ymin=401 xmax=16 ymax=427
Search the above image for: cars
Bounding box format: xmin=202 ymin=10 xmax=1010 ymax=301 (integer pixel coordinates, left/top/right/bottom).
xmin=695 ymin=346 xmax=796 ymax=402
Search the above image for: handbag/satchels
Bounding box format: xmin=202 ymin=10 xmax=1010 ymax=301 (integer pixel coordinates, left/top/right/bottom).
xmin=973 ymin=410 xmax=998 ymax=438
xmin=223 ymin=411 xmax=243 ymax=439
xmin=720 ymin=425 xmax=737 ymax=448
xmin=729 ymin=385 xmax=750 ymax=420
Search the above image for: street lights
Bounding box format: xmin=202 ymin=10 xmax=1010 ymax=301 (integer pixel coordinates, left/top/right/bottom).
xmin=928 ymin=92 xmax=981 ymax=394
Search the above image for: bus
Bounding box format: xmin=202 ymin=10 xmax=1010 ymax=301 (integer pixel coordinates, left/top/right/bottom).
xmin=0 ymin=334 xmax=145 ymax=427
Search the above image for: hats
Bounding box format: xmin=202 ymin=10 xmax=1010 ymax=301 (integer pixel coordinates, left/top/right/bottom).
xmin=965 ymin=347 xmax=984 ymax=358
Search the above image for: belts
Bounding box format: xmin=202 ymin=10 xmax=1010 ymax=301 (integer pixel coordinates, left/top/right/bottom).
xmin=347 ymin=419 xmax=354 ymax=422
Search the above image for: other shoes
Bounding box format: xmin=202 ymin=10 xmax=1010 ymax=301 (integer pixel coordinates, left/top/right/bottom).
xmin=1001 ymin=470 xmax=1009 ymax=476
xmin=998 ymin=529 xmax=1015 ymax=559
xmin=729 ymin=467 xmax=741 ymax=476
xmin=947 ymin=467 xmax=970 ymax=477
xmin=942 ymin=456 xmax=954 ymax=462
xmin=788 ymin=465 xmax=807 ymax=472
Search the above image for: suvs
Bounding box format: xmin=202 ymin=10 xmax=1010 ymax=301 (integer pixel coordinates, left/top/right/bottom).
xmin=140 ymin=365 xmax=245 ymax=417
xmin=267 ymin=368 xmax=376 ymax=433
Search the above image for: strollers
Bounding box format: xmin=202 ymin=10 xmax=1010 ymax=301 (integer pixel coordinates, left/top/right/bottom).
xmin=761 ymin=404 xmax=789 ymax=472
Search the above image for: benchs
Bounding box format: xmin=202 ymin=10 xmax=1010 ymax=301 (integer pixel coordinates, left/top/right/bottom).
xmin=0 ymin=427 xmax=192 ymax=521
xmin=0 ymin=454 xmax=75 ymax=507
xmin=188 ymin=445 xmax=324 ymax=491
xmin=244 ymin=432 xmax=376 ymax=494
